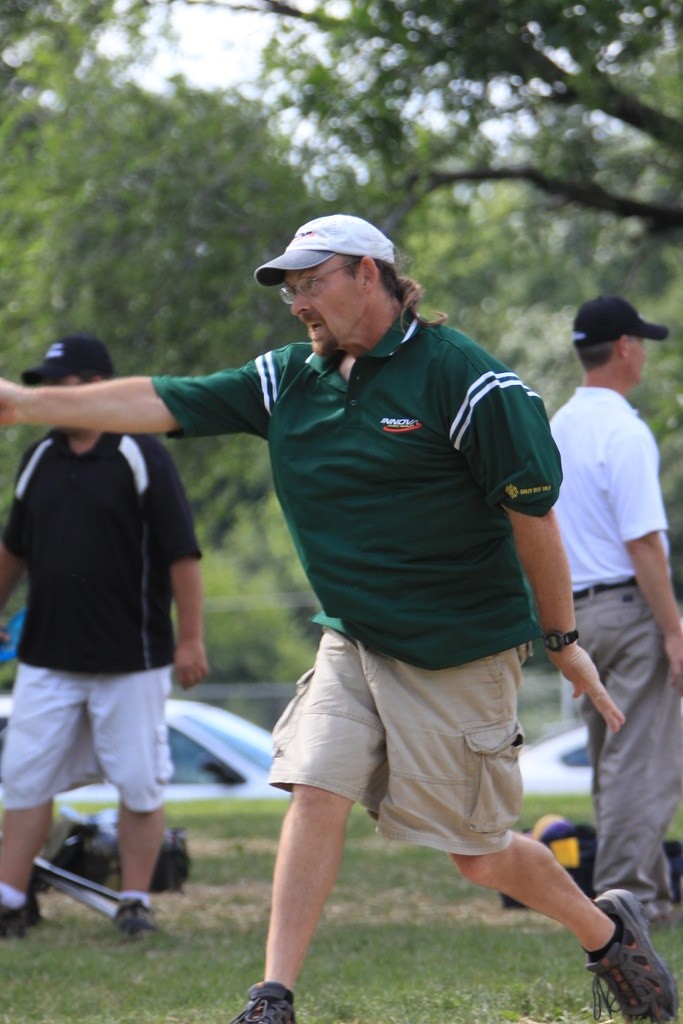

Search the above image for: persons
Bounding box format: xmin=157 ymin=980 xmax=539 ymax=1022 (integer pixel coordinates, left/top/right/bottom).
xmin=1 ymin=336 xmax=214 ymax=939
xmin=0 ymin=215 xmax=681 ymax=1024
xmin=547 ymin=294 xmax=682 ymax=930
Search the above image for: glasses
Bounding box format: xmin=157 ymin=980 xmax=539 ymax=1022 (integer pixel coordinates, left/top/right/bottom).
xmin=277 ymin=257 xmax=364 ymax=304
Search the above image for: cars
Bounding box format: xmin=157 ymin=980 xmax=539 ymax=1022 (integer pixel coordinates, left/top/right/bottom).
xmin=0 ymin=697 xmax=291 ymax=802
xmin=517 ymin=724 xmax=594 ymax=795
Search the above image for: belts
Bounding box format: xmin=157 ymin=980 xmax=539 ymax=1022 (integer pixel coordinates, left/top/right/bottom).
xmin=573 ymin=577 xmax=637 ymax=599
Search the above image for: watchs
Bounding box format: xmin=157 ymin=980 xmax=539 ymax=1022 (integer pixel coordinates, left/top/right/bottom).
xmin=542 ymin=629 xmax=580 ymax=652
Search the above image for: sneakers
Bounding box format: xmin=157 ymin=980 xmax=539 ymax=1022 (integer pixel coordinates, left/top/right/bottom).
xmin=585 ymin=888 xmax=678 ymax=1024
xmin=114 ymin=898 xmax=154 ymax=938
xmin=0 ymin=904 xmax=28 ymax=938
xmin=230 ymin=980 xmax=296 ymax=1024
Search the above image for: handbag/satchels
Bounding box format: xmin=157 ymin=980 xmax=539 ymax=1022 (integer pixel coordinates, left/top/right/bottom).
xmin=31 ymin=810 xmax=191 ymax=897
xmin=493 ymin=815 xmax=683 ymax=910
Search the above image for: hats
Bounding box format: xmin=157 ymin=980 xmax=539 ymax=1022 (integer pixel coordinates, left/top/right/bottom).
xmin=254 ymin=214 xmax=395 ymax=287
xmin=21 ymin=334 xmax=114 ymax=384
xmin=573 ymin=294 xmax=670 ymax=346
